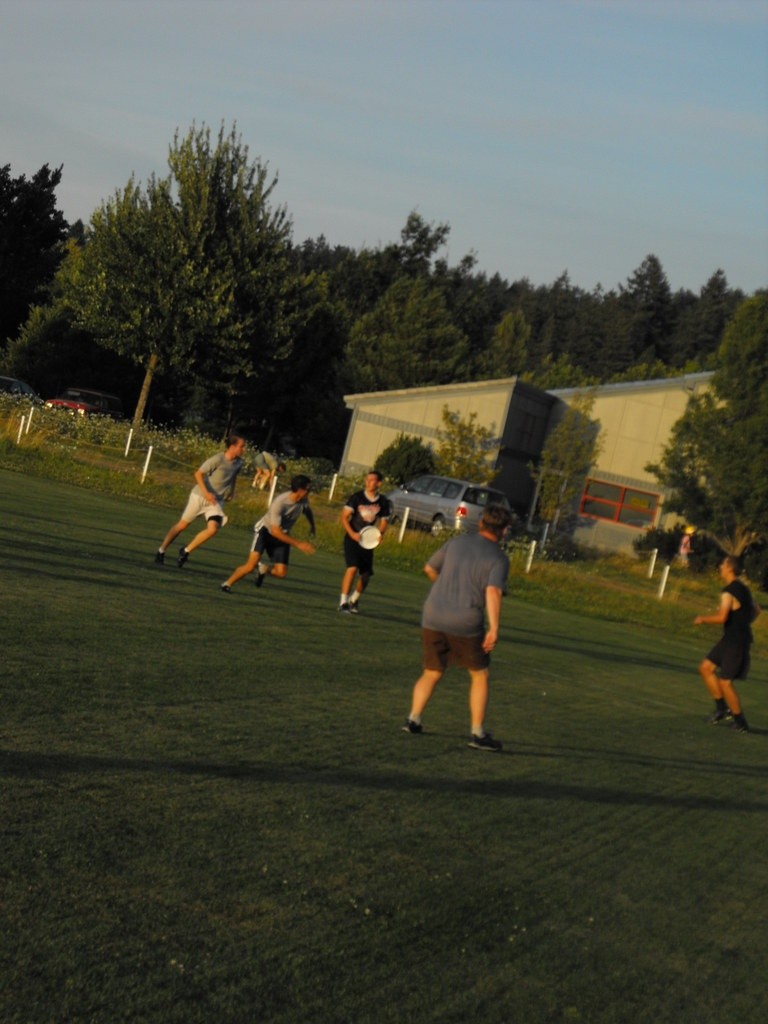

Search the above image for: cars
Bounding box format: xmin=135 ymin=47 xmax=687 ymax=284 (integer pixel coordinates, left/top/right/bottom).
xmin=44 ymin=398 xmax=98 ymax=418
xmin=0 ymin=375 xmax=44 ymax=407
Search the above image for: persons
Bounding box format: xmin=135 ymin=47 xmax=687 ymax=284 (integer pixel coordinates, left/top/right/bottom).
xmin=401 ymin=504 xmax=513 ymax=753
xmin=694 ymin=555 xmax=761 ymax=734
xmin=219 ymin=474 xmax=316 ymax=595
xmin=676 ymin=525 xmax=697 ymax=565
xmin=337 ymin=470 xmax=391 ymax=616
xmin=153 ymin=433 xmax=246 ymax=568
xmin=251 ymin=451 xmax=287 ymax=493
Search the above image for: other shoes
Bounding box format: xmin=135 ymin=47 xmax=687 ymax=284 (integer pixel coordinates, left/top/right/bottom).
xmin=707 ymin=710 xmax=732 ymax=725
xmin=255 ymin=569 xmax=265 ymax=588
xmin=468 ymin=733 xmax=502 ymax=751
xmin=348 ymin=597 xmax=359 ymax=614
xmin=155 ymin=550 xmax=164 ymax=565
xmin=221 ymin=582 xmax=231 ymax=594
xmin=728 ymin=723 xmax=747 ymax=733
xmin=402 ymin=719 xmax=422 ymax=733
xmin=178 ymin=547 xmax=189 ymax=567
xmin=337 ymin=602 xmax=351 ymax=614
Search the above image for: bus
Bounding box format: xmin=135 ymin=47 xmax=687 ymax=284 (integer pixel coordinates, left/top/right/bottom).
xmin=61 ymin=387 xmax=124 ymax=422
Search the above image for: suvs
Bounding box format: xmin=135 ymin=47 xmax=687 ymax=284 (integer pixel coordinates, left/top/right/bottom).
xmin=384 ymin=474 xmax=513 ymax=544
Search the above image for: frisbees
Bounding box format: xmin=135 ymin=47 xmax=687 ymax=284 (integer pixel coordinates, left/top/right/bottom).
xmin=358 ymin=525 xmax=382 ymax=550
